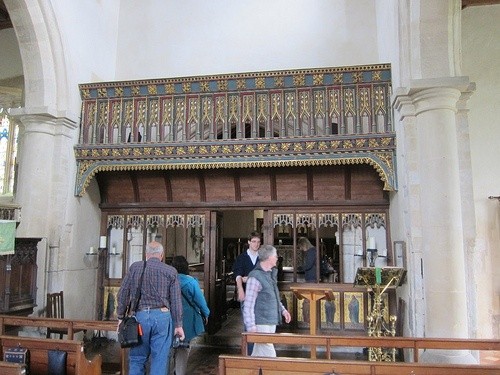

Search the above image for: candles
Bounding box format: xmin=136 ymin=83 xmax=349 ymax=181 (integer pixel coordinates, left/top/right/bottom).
xmin=91 ymin=235 xmax=116 ymax=254
xmin=358 ymin=237 xmax=388 ymax=256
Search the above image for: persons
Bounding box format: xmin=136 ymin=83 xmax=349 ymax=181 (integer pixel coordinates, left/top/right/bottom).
xmin=167 ymin=255 xmax=210 ymax=375
xmin=116 ymin=241 xmax=185 ymax=375
xmin=243 ymin=246 xmax=291 ymax=357
xmin=296 ymin=237 xmax=316 ymax=283
xmin=234 ymin=231 xmax=261 ymax=355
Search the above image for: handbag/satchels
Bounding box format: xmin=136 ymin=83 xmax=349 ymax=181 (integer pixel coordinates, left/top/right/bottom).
xmin=200 ymin=311 xmax=206 ymax=324
xmin=320 ymin=258 xmax=335 ymax=276
xmin=118 ymin=316 xmax=139 ymax=348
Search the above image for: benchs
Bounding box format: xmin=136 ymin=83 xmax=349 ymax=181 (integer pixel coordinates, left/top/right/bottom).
xmin=0 ymin=336 xmax=103 ymax=375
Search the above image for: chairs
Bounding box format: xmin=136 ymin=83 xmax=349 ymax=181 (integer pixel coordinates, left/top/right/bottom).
xmin=47 ymin=291 xmax=86 ymax=339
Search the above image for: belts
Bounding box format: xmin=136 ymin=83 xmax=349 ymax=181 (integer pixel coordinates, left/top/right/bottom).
xmin=138 ymin=305 xmax=167 ymax=311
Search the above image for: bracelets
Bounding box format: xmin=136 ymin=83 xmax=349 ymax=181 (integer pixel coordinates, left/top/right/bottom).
xmin=118 ymin=317 xmax=122 ymax=320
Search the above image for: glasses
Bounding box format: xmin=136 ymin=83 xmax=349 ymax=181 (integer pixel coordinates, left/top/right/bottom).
xmin=250 ymin=240 xmax=260 ymax=245
